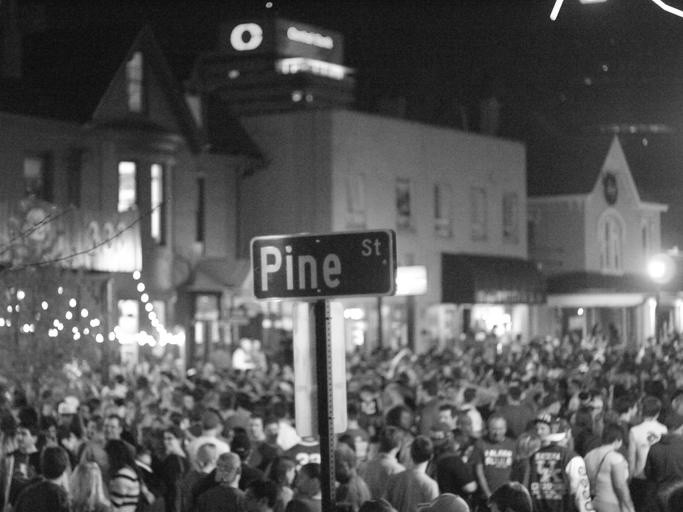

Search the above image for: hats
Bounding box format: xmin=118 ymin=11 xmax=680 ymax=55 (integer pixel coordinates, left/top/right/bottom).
xmin=532 ymin=412 xmax=561 ymax=433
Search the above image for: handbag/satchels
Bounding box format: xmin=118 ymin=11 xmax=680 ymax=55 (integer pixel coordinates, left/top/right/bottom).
xmin=138 ymin=483 xmax=155 ymax=506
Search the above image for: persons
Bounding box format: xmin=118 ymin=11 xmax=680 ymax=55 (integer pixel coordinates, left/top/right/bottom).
xmin=2 ymin=326 xmax=682 ymax=512
